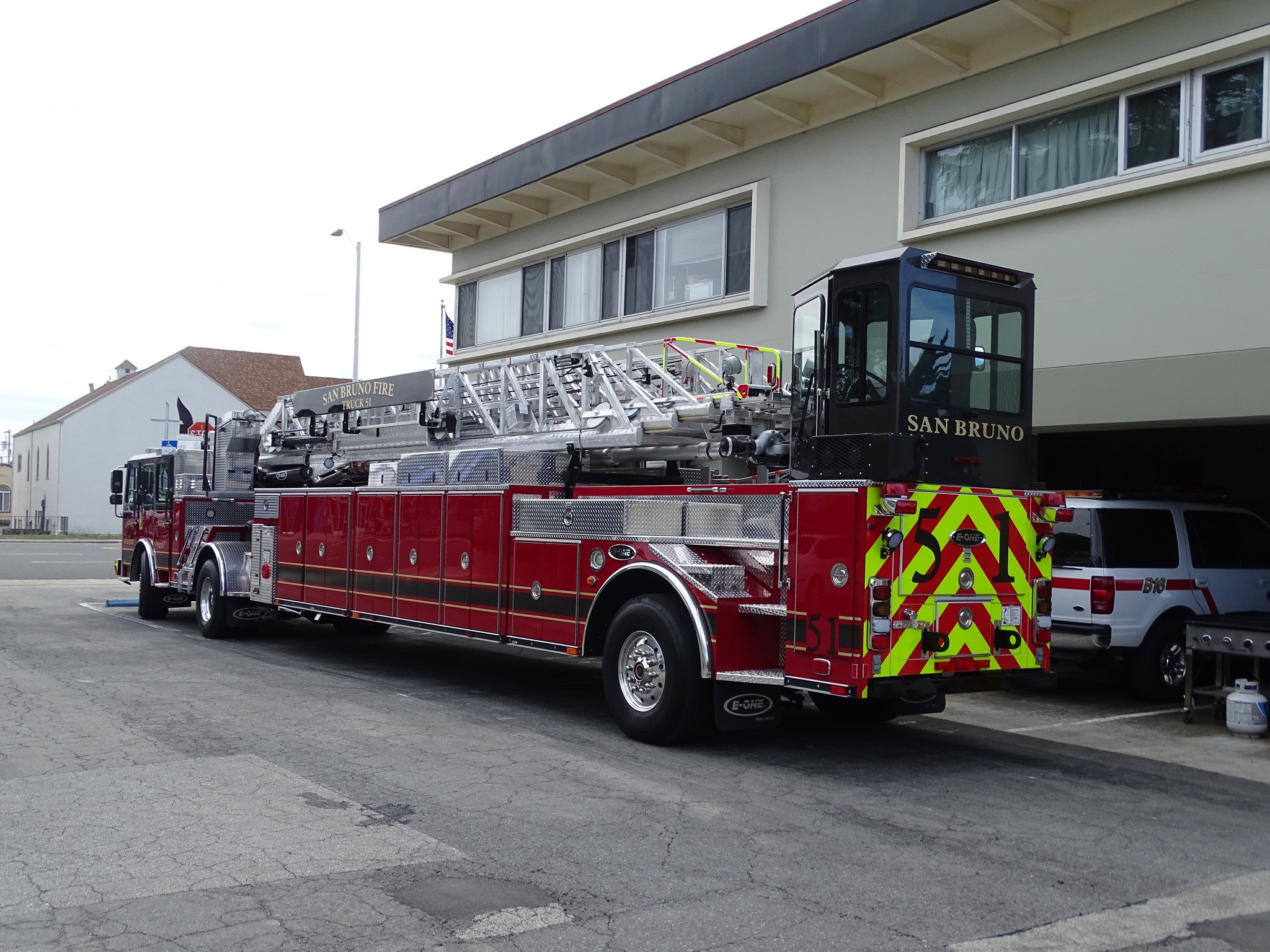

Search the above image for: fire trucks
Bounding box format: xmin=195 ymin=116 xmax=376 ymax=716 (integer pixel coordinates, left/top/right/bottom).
xmin=105 ymin=247 xmax=1053 ymax=746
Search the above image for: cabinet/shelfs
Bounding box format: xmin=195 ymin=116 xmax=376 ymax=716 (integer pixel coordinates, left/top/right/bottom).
xmin=685 ymin=282 xmax=717 ymax=301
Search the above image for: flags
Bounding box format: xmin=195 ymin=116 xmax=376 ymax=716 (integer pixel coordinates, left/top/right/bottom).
xmin=443 ymin=311 xmax=454 ymax=355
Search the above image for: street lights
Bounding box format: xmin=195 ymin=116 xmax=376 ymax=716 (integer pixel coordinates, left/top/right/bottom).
xmin=331 ymin=228 xmax=361 ymax=383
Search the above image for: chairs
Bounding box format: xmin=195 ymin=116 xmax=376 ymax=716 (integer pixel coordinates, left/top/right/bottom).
xmin=880 ymin=310 xmax=976 ymax=402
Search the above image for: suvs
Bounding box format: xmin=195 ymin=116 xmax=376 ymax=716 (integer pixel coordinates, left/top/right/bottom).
xmin=1051 ymin=489 xmax=1270 ymax=702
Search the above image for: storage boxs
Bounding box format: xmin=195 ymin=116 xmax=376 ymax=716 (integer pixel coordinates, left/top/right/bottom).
xmin=686 ymin=274 xmax=701 ymax=285
